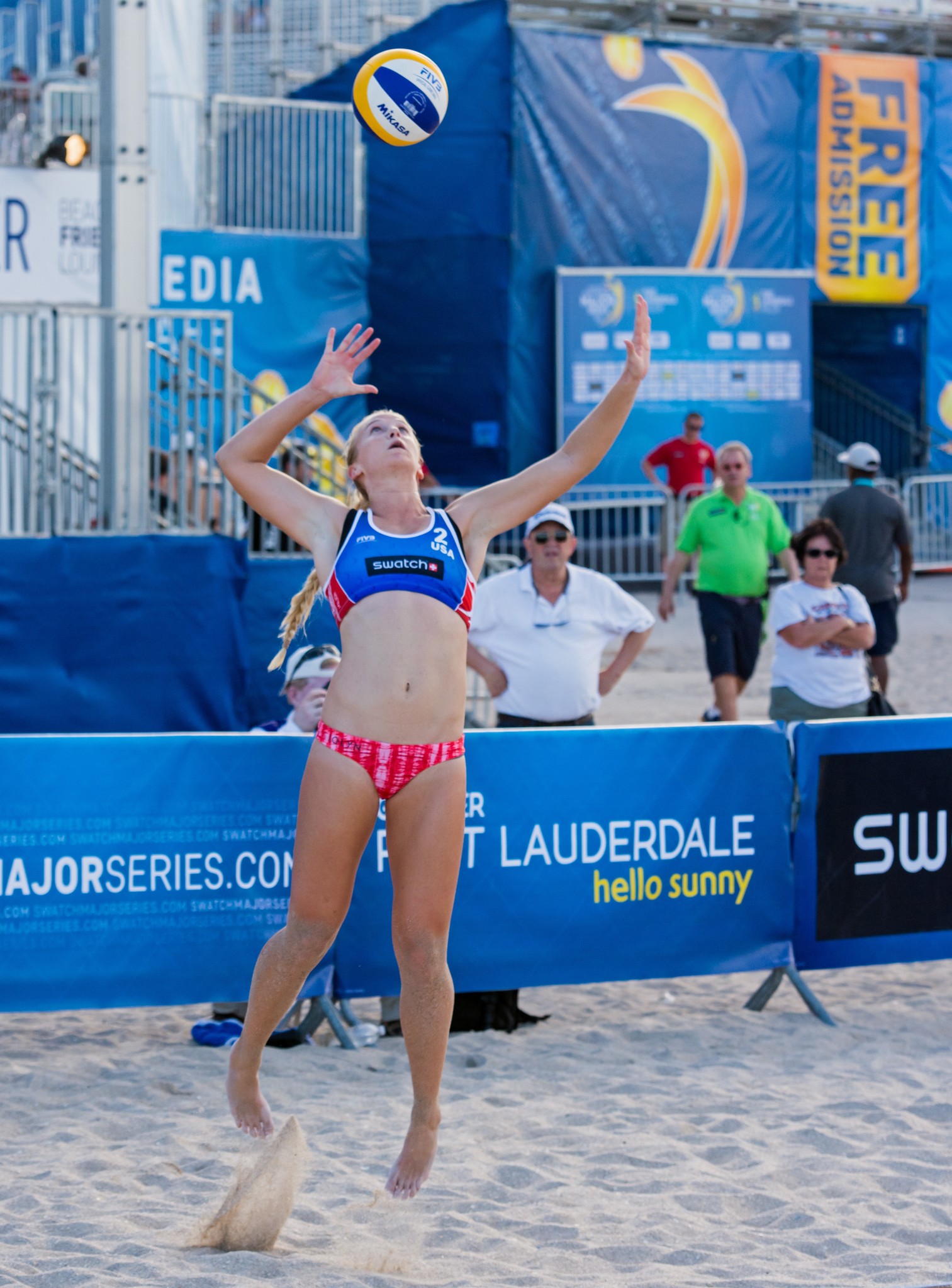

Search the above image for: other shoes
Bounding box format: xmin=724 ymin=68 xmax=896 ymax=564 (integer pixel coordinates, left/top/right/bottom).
xmin=701 ymin=710 xmax=721 ymax=722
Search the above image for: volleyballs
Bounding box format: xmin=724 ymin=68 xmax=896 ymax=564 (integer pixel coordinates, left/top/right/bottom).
xmin=351 ymin=48 xmax=449 ymax=146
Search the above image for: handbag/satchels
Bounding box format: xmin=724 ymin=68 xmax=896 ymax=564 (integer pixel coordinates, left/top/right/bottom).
xmin=868 ymin=689 xmax=895 ymax=717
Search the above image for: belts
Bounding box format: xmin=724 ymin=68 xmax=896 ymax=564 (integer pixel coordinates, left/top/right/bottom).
xmin=667 ymin=493 xmax=697 ymax=503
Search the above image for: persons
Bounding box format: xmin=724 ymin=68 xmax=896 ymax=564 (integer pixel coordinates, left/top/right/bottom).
xmin=464 ymin=501 xmax=652 ymax=734
xmin=78 ymin=431 xmax=321 ymax=558
xmin=650 ymin=439 xmax=798 ymax=730
xmin=647 ymin=409 xmax=719 ymax=595
xmin=819 ymin=443 xmax=912 ymax=697
xmin=767 ymin=513 xmax=878 ymax=733
xmin=212 ymin=290 xmax=653 ymax=1201
xmin=204 ymin=638 xmax=338 ymax=1048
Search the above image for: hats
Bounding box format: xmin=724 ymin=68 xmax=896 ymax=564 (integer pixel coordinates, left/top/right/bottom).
xmin=836 ymin=442 xmax=881 ymax=472
xmin=524 ymin=502 xmax=575 ymax=539
xmin=278 ymin=643 xmax=342 ymax=696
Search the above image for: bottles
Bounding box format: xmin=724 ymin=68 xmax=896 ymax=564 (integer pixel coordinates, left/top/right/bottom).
xmin=325 ymin=1023 xmax=386 ymax=1047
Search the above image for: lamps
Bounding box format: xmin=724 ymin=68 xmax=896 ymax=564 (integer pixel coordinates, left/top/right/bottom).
xmin=37 ymin=134 xmax=93 ymax=171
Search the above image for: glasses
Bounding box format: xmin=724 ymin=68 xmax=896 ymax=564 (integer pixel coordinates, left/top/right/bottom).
xmin=535 ymin=531 xmax=567 ymax=545
xmin=288 ymin=646 xmax=336 ymax=683
xmin=806 ymin=548 xmax=837 ymax=559
xmin=724 ymin=462 xmax=741 ymax=471
xmin=688 ymin=425 xmax=702 ymax=431
